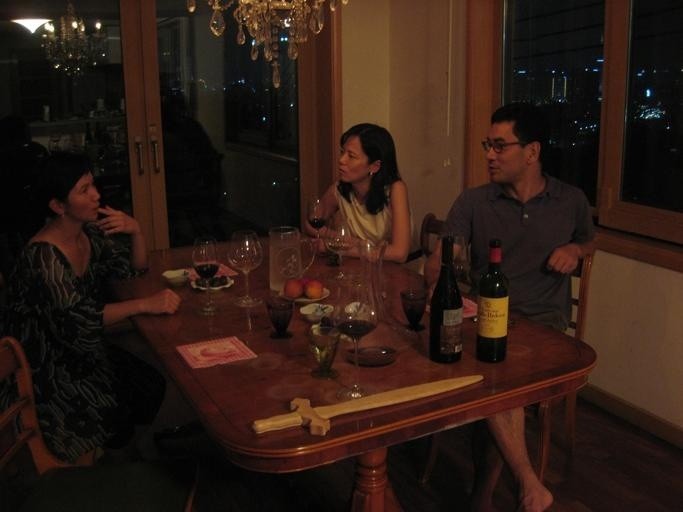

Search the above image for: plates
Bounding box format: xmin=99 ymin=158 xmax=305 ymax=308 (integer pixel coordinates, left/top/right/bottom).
xmin=186 ymin=275 xmax=233 ymax=291
xmin=278 ymin=287 xmax=330 ymax=304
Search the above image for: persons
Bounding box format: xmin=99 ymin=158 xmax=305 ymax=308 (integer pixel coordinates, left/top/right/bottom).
xmin=304 ymin=123 xmax=415 ymax=265
xmin=425 ymin=103 xmax=597 ymax=511
xmin=8 ymin=159 xmax=182 ymax=462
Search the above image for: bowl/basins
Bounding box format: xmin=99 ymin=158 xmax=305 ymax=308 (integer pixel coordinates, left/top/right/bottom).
xmin=161 ymin=269 xmax=190 ymax=287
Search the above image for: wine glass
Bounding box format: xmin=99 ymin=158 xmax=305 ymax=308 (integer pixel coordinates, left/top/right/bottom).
xmin=191 ymin=234 xmax=223 ymax=316
xmin=326 ymin=217 xmax=355 ymax=281
xmin=306 ymin=195 xmax=325 ymax=256
xmin=226 ymin=231 xmax=264 ymax=307
xmin=336 ymin=272 xmax=380 ymax=402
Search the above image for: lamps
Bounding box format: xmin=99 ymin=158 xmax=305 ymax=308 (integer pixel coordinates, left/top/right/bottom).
xmin=177 ymin=0 xmax=357 ymax=88
xmin=40 ymin=0 xmax=108 ymax=78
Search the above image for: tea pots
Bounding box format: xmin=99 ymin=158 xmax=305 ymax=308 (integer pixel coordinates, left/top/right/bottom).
xmin=267 ymin=226 xmax=314 ymax=292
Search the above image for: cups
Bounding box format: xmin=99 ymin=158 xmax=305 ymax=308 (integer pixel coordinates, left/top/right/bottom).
xmin=303 ymin=326 xmax=338 ymax=379
xmin=398 ymin=287 xmax=425 ymax=331
xmin=264 ymin=298 xmax=293 ymax=340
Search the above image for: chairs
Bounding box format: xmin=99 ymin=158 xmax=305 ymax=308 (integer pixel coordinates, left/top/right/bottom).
xmin=0 ymin=335 xmax=203 ymax=512
xmin=414 ymin=236 xmax=591 ymax=488
xmin=419 ymin=212 xmax=444 ymax=250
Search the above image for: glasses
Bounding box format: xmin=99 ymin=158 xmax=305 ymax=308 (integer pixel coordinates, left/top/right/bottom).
xmin=482 ymin=140 xmax=528 ymax=152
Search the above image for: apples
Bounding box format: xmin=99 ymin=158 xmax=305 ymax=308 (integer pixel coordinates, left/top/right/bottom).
xmin=301 ymin=277 xmax=311 ymax=286
xmin=304 ymin=280 xmax=324 ymax=299
xmin=284 ymin=278 xmax=304 ymax=299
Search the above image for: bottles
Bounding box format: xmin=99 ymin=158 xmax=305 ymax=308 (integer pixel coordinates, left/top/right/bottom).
xmin=472 ymin=237 xmax=508 ymax=361
xmin=338 ymin=238 xmax=413 ymax=368
xmin=427 ymin=236 xmax=463 ymax=364
